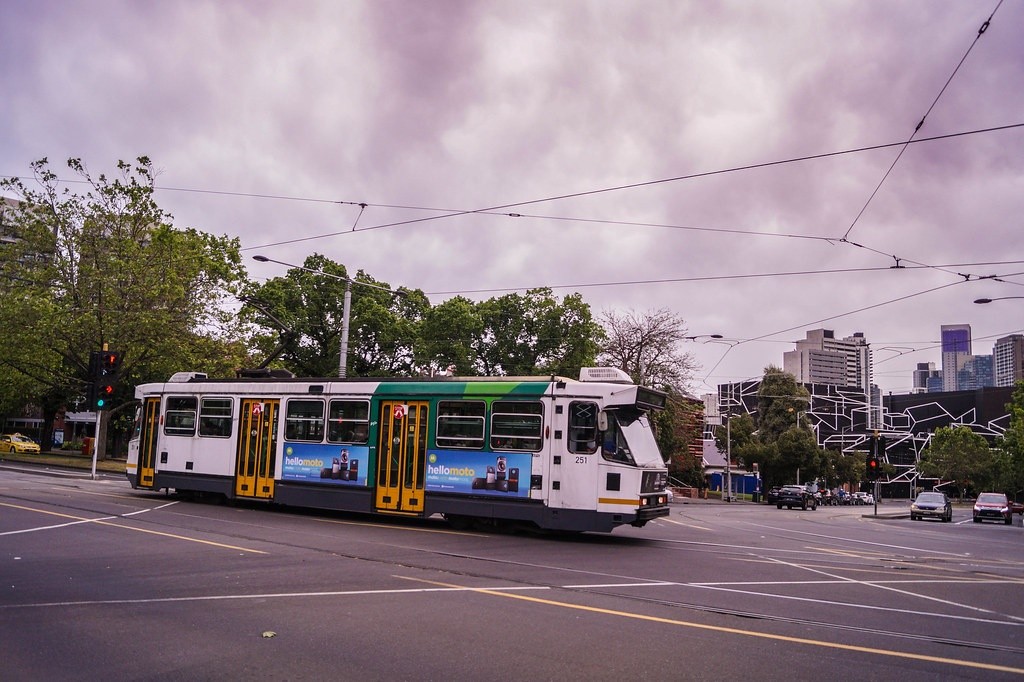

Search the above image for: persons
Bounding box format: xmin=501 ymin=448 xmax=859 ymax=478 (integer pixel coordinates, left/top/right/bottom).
xmin=837 ymin=488 xmax=847 ymax=499
xmin=585 ymin=424 xmax=617 ymax=457
xmin=702 ymin=478 xmax=710 ymax=499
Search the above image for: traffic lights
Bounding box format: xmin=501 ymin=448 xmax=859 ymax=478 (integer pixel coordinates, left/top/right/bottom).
xmin=86 ymin=350 xmax=118 ymax=410
xmin=866 ymin=458 xmax=879 ymax=480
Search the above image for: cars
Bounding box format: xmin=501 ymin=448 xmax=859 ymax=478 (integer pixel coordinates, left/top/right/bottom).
xmin=910 ymin=491 xmax=952 ymax=522
xmin=817 ymin=490 xmax=873 ymax=507
xmin=1012 ymin=503 xmax=1024 ymax=515
xmin=0 ymin=432 xmax=40 ymax=454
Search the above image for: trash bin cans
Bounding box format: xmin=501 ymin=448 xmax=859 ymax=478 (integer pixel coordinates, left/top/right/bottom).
xmin=752 ymin=491 xmax=761 ymax=502
xmin=81 ymin=437 xmax=95 ymax=455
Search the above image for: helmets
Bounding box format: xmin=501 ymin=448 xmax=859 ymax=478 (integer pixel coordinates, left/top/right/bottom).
xmin=840 ymin=488 xmax=843 ymax=491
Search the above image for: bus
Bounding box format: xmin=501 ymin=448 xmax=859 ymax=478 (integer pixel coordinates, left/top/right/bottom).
xmin=126 ymin=366 xmax=670 ymax=536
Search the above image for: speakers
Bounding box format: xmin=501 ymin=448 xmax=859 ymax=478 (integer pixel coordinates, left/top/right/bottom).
xmin=472 ymin=457 xmax=519 ymax=493
xmin=321 ymin=448 xmax=359 ymax=482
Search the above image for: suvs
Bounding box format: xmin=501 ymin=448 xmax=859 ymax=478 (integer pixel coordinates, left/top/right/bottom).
xmin=777 ymin=484 xmax=817 ymax=510
xmin=973 ymin=492 xmax=1013 ymax=525
xmin=768 ymin=486 xmax=780 ymax=505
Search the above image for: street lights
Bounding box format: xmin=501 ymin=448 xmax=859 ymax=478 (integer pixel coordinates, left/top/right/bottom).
xmin=253 ymin=255 xmax=408 ymax=379
